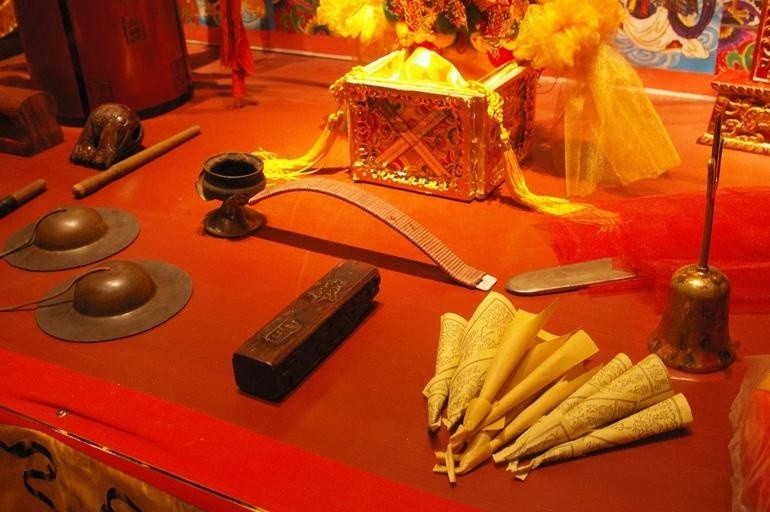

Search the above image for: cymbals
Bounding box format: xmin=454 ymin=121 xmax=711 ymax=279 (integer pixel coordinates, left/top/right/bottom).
xmin=35 ymin=260 xmax=193 ymax=343
xmin=3 ymin=205 xmax=140 ymax=271
